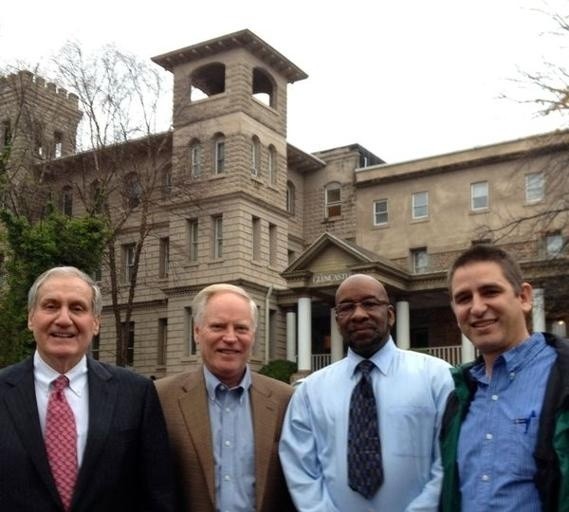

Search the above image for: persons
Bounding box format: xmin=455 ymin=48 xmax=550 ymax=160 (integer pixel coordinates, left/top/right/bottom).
xmin=0 ymin=265 xmax=175 ymax=511
xmin=438 ymin=244 xmax=569 ymax=510
xmin=274 ymin=272 xmax=456 ymax=511
xmin=150 ymin=281 xmax=294 ymax=510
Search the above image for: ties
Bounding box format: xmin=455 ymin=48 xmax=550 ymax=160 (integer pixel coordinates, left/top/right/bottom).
xmin=43 ymin=375 xmax=78 ymax=510
xmin=348 ymin=360 xmax=383 ymax=499
